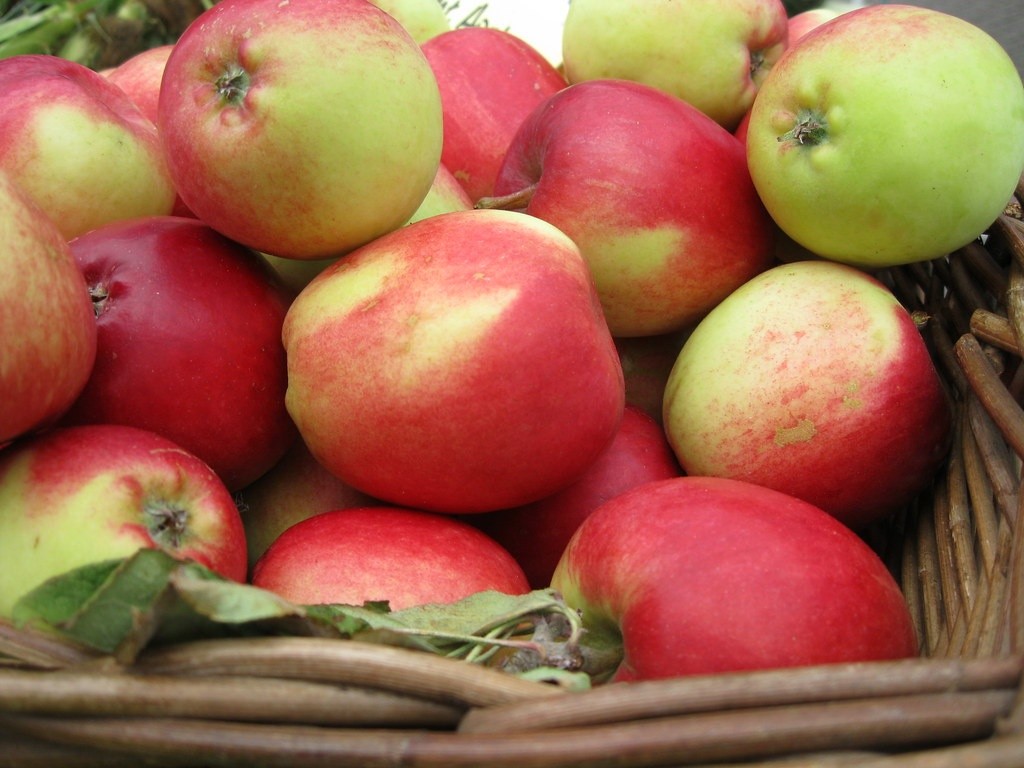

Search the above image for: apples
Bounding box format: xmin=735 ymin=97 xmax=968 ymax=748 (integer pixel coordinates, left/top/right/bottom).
xmin=0 ymin=0 xmax=1024 ymax=685
